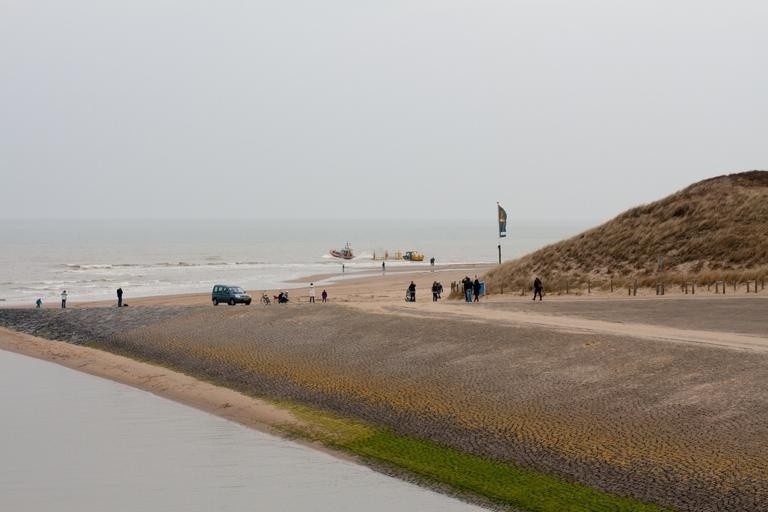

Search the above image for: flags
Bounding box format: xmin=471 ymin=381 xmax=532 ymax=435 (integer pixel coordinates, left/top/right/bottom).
xmin=499 ymin=204 xmax=508 ymax=238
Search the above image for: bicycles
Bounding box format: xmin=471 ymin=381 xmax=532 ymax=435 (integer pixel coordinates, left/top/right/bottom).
xmin=260 ymin=294 xmax=270 ymax=304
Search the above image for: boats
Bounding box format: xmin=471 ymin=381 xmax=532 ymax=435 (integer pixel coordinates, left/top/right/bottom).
xmin=371 ymin=251 xmax=402 ymax=261
xmin=403 ymin=250 xmax=424 ymax=261
xmin=330 ymin=241 xmax=354 ymax=259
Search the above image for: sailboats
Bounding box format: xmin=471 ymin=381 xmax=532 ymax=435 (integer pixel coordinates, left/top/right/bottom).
xmin=497 ymin=201 xmax=507 ymax=237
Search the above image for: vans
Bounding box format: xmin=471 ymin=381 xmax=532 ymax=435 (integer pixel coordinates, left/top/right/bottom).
xmin=212 ymin=284 xmax=251 ymax=305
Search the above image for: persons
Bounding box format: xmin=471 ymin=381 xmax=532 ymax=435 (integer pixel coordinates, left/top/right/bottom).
xmin=531 ymin=277 xmax=543 ymax=301
xmin=116 ymin=287 xmax=124 ymax=307
xmin=60 ymin=289 xmax=68 ymax=307
xmin=283 ymin=290 xmax=290 ymax=301
xmin=36 ymin=298 xmax=42 ymax=307
xmin=407 ymin=274 xmax=482 ymax=303
xmin=278 ymin=292 xmax=289 ymax=303
xmin=321 ymin=288 xmax=328 ymax=303
xmin=308 ymin=282 xmax=316 ymax=303
